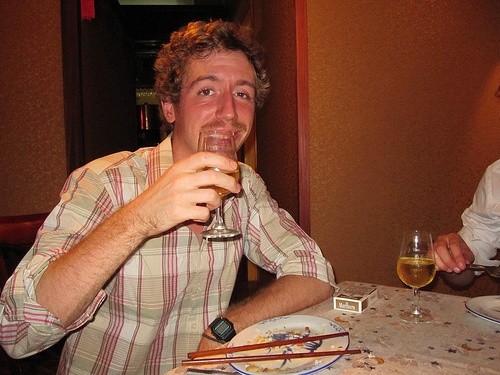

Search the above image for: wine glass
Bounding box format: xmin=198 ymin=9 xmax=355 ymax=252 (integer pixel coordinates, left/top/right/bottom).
xmin=397 ymin=231 xmax=436 ymax=321
xmin=197 ymin=131 xmax=240 ymax=238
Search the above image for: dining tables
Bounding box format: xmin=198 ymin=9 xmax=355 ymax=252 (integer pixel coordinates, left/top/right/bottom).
xmin=163 ymin=281 xmax=499 ymax=375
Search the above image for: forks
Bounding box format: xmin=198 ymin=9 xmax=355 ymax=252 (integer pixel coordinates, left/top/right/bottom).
xmin=466 ymin=264 xmax=500 ymax=277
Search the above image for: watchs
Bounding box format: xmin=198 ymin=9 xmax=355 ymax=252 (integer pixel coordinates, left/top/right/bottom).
xmin=208 ymin=316 xmax=236 ymax=342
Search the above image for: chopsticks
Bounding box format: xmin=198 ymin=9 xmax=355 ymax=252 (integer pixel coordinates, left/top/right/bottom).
xmin=182 ymin=332 xmax=361 ymax=365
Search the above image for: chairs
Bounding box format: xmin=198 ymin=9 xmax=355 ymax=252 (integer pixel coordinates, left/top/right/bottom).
xmin=0 ymin=212 xmax=68 ymax=375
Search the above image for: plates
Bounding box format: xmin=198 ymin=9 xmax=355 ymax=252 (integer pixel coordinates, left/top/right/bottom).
xmin=227 ymin=314 xmax=350 ymax=375
xmin=464 ymin=295 xmax=500 ymax=324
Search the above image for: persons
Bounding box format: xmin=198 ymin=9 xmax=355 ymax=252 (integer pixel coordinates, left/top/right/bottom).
xmin=434 ymin=158 xmax=500 ymax=289
xmin=1 ymin=20 xmax=341 ymax=374
